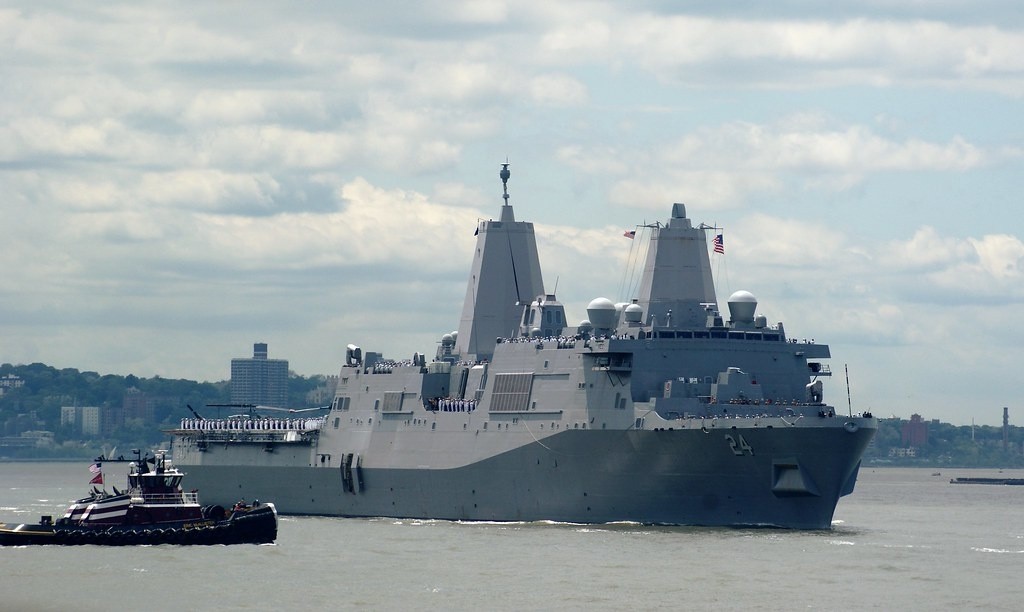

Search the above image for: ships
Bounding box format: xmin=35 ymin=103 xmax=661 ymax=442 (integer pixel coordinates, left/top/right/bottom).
xmin=156 ymin=154 xmax=878 ymax=530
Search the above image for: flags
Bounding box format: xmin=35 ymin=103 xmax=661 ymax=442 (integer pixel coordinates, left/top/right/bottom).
xmin=88 ymin=462 xmax=102 ymax=485
xmin=623 ymin=230 xmax=635 ymax=239
xmin=711 ymin=235 xmax=724 ymax=254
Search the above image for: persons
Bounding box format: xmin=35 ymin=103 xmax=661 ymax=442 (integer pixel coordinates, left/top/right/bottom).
xmin=344 ymin=360 xmax=490 ymax=374
xmin=428 ymin=396 xmax=478 ymax=411
xmin=673 ymin=395 xmax=873 ymax=419
xmin=179 ymin=417 xmax=326 ymax=429
xmin=788 ymin=337 xmax=816 ymax=344
xmin=496 ymin=331 xmax=629 ymax=350
xmin=233 ymin=497 xmax=247 ymax=512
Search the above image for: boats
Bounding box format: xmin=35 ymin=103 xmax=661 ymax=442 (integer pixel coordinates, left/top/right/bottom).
xmin=0 ymin=446 xmax=280 ymax=546
